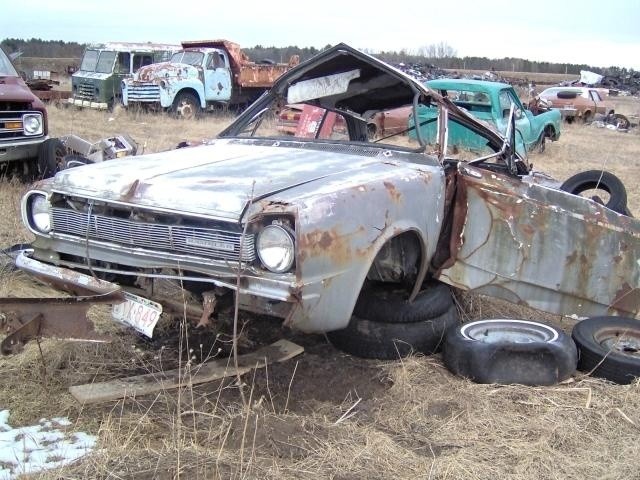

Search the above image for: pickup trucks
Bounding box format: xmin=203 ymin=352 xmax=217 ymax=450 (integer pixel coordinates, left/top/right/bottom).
xmin=524 ymin=86 xmax=638 ymax=130
xmin=405 ymin=78 xmax=562 ymax=164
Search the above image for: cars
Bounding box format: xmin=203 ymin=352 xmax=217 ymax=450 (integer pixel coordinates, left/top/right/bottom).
xmin=2 ymin=42 xmax=640 ymax=339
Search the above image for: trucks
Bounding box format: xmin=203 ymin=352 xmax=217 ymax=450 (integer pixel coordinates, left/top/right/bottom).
xmin=119 ymin=42 xmax=300 ymax=121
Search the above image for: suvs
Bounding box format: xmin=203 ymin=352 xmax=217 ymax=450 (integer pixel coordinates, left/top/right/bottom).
xmin=2 ymin=49 xmax=49 ymax=168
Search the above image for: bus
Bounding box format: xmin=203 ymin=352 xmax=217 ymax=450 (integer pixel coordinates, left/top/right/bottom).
xmin=65 ymin=44 xmax=184 ymax=111
